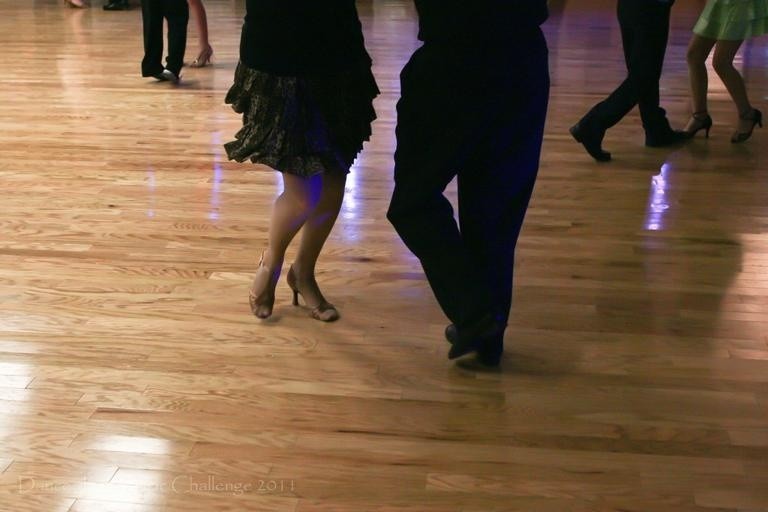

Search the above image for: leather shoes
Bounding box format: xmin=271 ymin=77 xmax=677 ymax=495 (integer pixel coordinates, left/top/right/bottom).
xmin=142 ymin=64 xmax=179 ymax=80
xmin=446 ymin=321 xmax=505 ymax=371
xmin=568 ymin=122 xmax=611 ymax=160
xmin=647 ymin=126 xmax=690 ymax=147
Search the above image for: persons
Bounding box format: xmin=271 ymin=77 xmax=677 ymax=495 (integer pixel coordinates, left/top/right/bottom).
xmin=139 ymin=1 xmax=189 ymax=84
xmin=61 ymin=0 xmax=92 ymax=10
xmin=223 ymin=0 xmax=380 ymax=323
xmin=682 ymin=1 xmax=768 ymax=143
xmin=568 ymin=127 xmax=752 ymax=386
xmin=384 ymin=1 xmax=552 ymax=367
xmin=162 ymin=0 xmax=213 ymax=67
xmin=568 ymin=1 xmax=694 ymax=163
xmin=101 ymin=0 xmax=131 ymax=11
xmin=57 ymin=7 xmax=96 ymax=156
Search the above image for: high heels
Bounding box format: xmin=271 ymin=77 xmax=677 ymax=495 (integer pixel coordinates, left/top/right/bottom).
xmin=287 ymin=266 xmax=338 ymax=321
xmin=249 ymin=248 xmax=282 ymax=317
xmin=681 ymin=112 xmax=712 ymax=140
xmin=192 ymin=47 xmax=212 ymax=67
xmin=731 ymin=109 xmax=763 ymax=143
